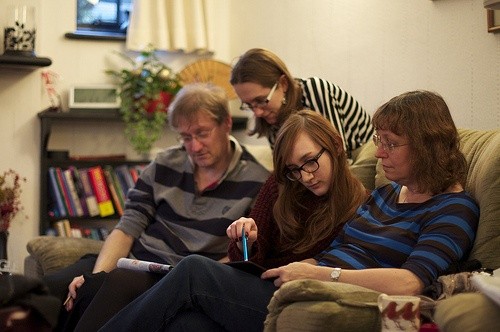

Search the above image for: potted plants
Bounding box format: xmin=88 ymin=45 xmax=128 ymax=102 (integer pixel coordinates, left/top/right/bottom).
xmin=101 ymin=38 xmax=188 ymax=159
xmin=0 ymin=169 xmax=30 ymax=268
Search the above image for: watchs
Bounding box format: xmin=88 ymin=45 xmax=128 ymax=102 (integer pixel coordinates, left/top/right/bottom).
xmin=329 ymin=267 xmax=341 ymax=281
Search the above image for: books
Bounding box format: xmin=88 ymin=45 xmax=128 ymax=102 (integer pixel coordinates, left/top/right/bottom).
xmin=46 ymin=162 xmax=145 ymax=242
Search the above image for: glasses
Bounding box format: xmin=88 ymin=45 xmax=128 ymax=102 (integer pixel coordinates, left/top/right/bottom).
xmin=372 ymin=133 xmax=411 ymax=155
xmin=175 ymin=119 xmax=226 ymax=147
xmin=285 ymin=148 xmax=327 ymax=182
xmin=238 ymin=82 xmax=280 ymax=113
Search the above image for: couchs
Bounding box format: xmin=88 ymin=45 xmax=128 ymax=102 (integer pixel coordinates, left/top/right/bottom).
xmin=23 ymin=127 xmax=500 ymax=332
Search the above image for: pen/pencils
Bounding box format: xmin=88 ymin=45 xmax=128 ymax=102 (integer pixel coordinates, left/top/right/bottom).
xmin=242 ymin=226 xmax=248 ymax=262
xmin=63 ymin=274 xmax=84 ymax=306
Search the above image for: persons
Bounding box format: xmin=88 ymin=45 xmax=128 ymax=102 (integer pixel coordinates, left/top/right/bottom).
xmin=230 ymin=49 xmax=377 ymax=160
xmin=0 ymin=83 xmax=275 ymax=331
xmin=224 ymin=108 xmax=369 ymax=274
xmin=96 ymin=88 xmax=479 ymax=332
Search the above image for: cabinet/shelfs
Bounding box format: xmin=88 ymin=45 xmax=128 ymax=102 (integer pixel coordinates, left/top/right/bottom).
xmin=36 ymin=105 xmax=251 ymax=242
